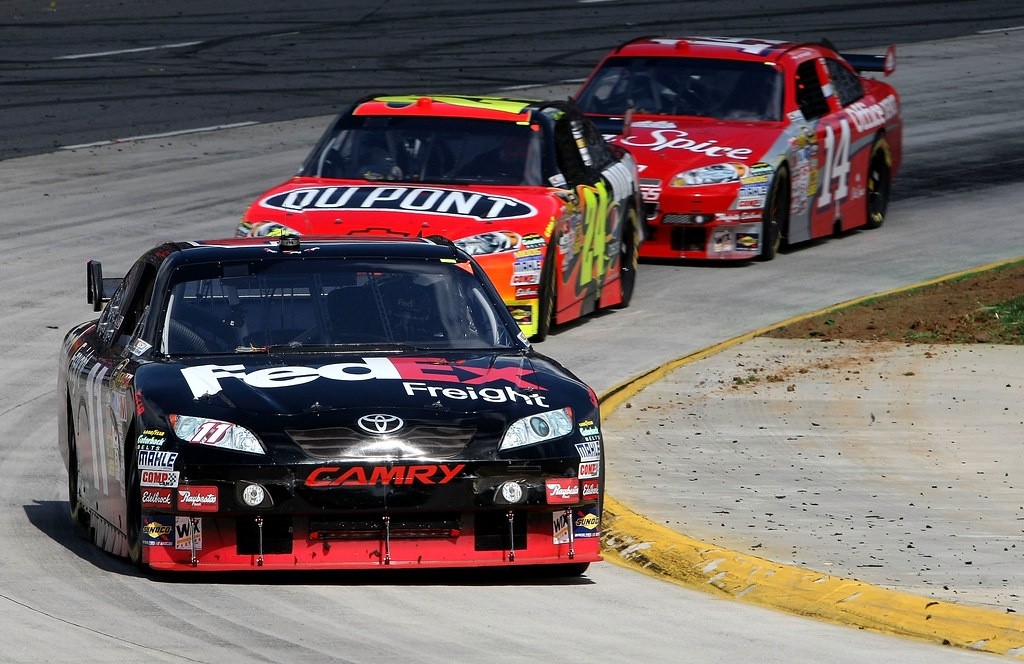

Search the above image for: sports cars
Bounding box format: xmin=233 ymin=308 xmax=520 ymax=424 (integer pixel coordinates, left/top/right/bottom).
xmin=566 ymin=32 xmax=904 ymax=266
xmin=232 ymin=90 xmax=645 ymax=345
xmin=53 ymin=227 xmax=608 ymax=580
